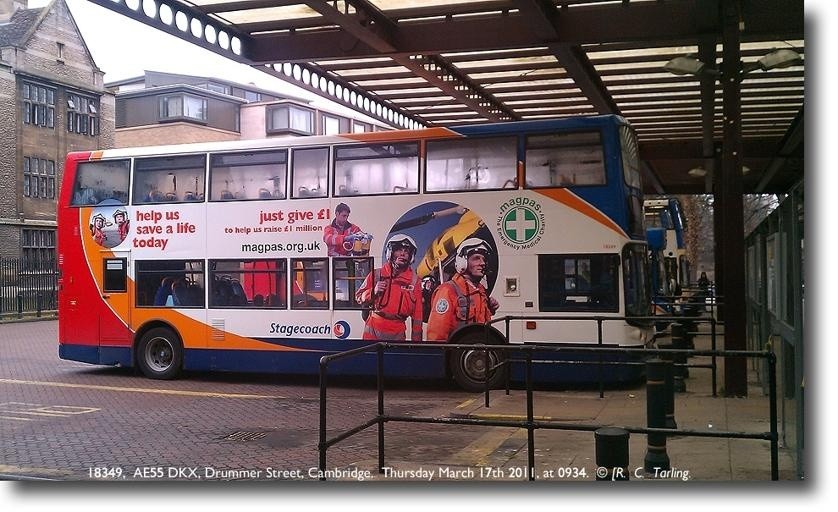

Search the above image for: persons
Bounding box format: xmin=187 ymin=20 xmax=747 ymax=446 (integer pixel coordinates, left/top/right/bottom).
xmin=697 ymin=271 xmax=708 ymax=287
xmin=425 ymin=236 xmax=501 ymax=340
xmin=322 ymin=202 xmax=374 ymax=257
xmin=667 ymin=272 xmax=677 ymax=296
xmin=111 ymin=208 xmax=130 ymax=239
xmin=421 ymin=274 xmax=439 ymax=322
xmin=85 ymin=214 xmax=109 ymax=245
xmin=353 ymin=233 xmax=425 ymax=340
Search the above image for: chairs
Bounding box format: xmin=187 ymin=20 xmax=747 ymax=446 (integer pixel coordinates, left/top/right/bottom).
xmin=69 ymin=184 xmax=376 ymax=312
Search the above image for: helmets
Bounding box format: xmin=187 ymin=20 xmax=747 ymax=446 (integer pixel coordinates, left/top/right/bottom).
xmin=113 ymin=209 xmax=127 ymax=224
xmin=455 ymin=238 xmax=492 ymax=274
xmin=385 ymin=233 xmax=417 ymax=264
xmin=91 ymin=213 xmax=106 ymax=226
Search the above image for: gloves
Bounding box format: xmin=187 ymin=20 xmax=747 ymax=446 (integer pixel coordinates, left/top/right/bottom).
xmin=344 ymin=232 xmax=373 ymax=240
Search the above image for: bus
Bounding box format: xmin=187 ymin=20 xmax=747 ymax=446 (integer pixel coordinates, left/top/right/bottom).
xmin=52 ymin=112 xmax=658 ymax=395
xmin=642 ymin=196 xmax=690 ymax=298
